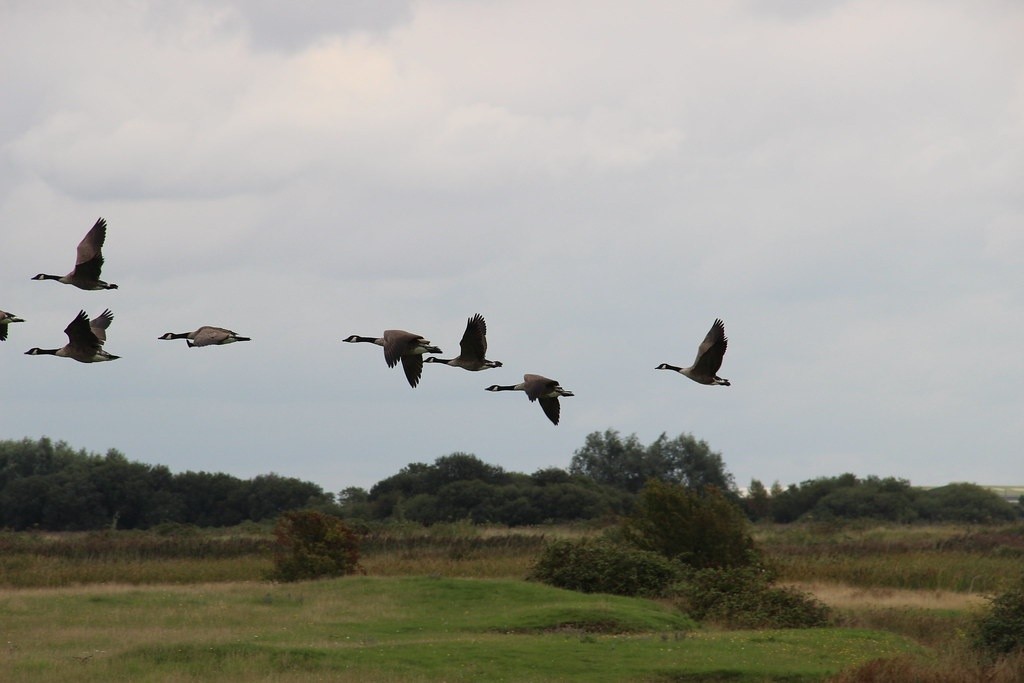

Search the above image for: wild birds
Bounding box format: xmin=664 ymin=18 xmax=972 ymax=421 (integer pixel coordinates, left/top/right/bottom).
xmin=341 ymin=312 xmax=503 ymax=389
xmin=484 ymin=374 xmax=575 ymax=426
xmin=654 ymin=318 xmax=731 ymax=387
xmin=31 ymin=216 xmax=119 ymax=291
xmin=0 ymin=309 xmax=27 ymax=342
xmin=24 ymin=309 xmax=123 ymax=363
xmin=157 ymin=325 xmax=251 ymax=348
